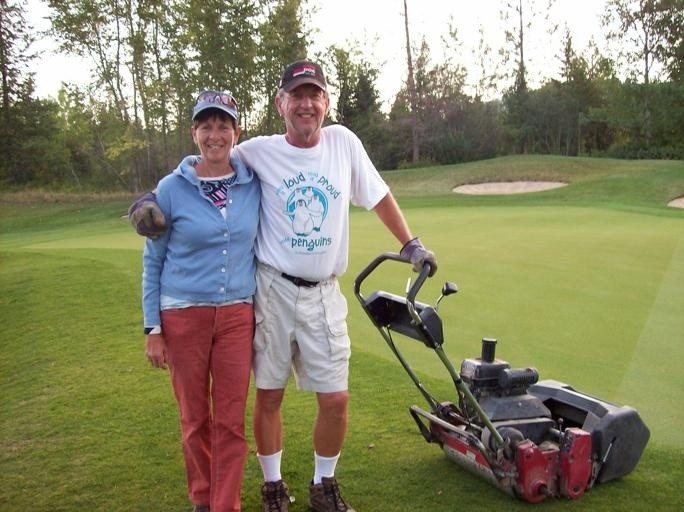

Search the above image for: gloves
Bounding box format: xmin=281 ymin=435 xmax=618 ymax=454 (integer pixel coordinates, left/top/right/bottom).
xmin=128 ymin=191 xmax=168 ymax=241
xmin=400 ymin=236 xmax=438 ymax=278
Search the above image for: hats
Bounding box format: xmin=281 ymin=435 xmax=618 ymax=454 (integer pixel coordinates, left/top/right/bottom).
xmin=278 ymin=60 xmax=326 ymax=92
xmin=191 ymin=90 xmax=238 ymax=121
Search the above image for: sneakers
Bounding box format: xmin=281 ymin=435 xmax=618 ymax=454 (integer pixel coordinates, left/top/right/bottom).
xmin=308 ymin=476 xmax=357 ymax=512
xmin=261 ymin=479 xmax=289 ymax=511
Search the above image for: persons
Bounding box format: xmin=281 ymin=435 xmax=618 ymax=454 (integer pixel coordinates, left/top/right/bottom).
xmin=128 ymin=60 xmax=438 ymax=512
xmin=138 ymin=90 xmax=262 ymax=512
